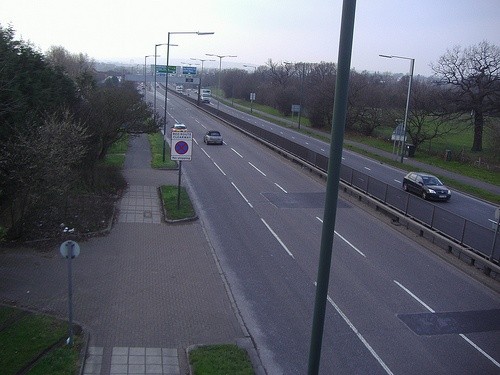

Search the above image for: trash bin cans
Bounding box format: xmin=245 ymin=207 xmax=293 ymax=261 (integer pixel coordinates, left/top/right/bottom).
xmin=406 ymin=144 xmax=414 ymax=158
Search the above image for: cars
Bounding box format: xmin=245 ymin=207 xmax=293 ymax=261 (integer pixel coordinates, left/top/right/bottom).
xmin=171 ymin=123 xmax=187 ymax=132
xmin=402 ymin=172 xmax=451 ymax=201
xmin=204 ymin=131 xmax=223 ymax=145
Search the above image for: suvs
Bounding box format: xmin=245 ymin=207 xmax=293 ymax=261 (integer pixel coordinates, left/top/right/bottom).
xmin=201 ymin=93 xmax=211 ymax=103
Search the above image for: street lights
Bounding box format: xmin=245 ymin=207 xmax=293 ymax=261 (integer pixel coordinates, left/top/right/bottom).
xmin=379 ymin=54 xmax=415 ymax=155
xmin=205 ymin=54 xmax=237 ymax=115
xmin=191 ymin=58 xmax=216 ymax=106
xmin=145 ymin=55 xmax=161 ymax=110
xmin=243 ymin=65 xmax=257 ymax=113
xmin=162 ymin=31 xmax=215 ymax=163
xmin=154 ymin=43 xmax=178 ymax=118
xmin=283 ymin=61 xmax=304 ymax=130
